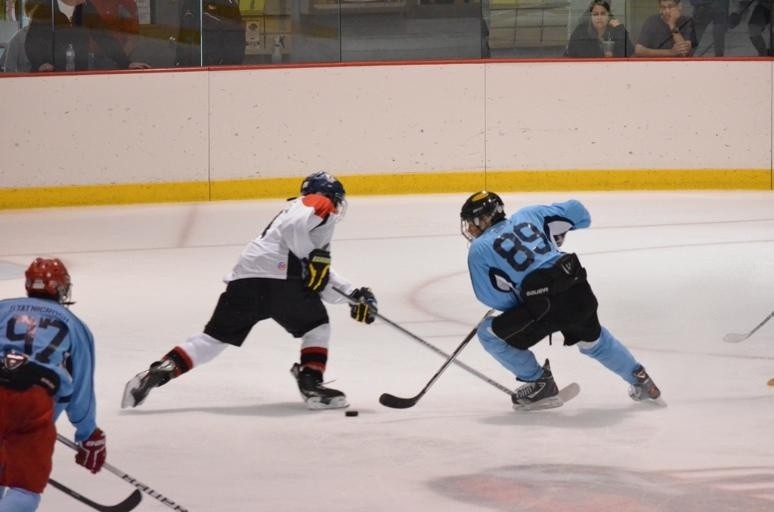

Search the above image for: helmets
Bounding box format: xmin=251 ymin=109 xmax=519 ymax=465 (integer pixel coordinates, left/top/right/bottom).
xmin=457 ymin=191 xmax=506 ymax=241
xmin=23 ymin=256 xmax=71 ymax=305
xmin=301 ymin=172 xmax=347 ymax=222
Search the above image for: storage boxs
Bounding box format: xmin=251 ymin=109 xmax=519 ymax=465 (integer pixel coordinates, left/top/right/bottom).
xmin=238 ymin=0 xmax=292 ymax=16
xmin=241 ymin=16 xmax=293 ymax=34
xmin=243 ymin=32 xmax=293 ymax=55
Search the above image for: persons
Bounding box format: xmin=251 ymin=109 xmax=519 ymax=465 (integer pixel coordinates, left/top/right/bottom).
xmin=131 ymin=171 xmax=378 ymax=406
xmin=687 ymin=0 xmax=730 ymax=57
xmin=634 ymin=0 xmax=697 ymax=57
xmin=0 ymin=258 xmax=108 ymax=512
xmin=3 ymin=0 xmax=39 ymax=73
xmin=25 ymin=0 xmax=152 ymax=73
xmin=562 ymin=0 xmax=634 ymax=58
xmin=175 ymin=0 xmax=246 ymax=65
xmin=460 ymin=191 xmax=660 ymax=405
xmin=88 ymin=0 xmax=139 ymax=67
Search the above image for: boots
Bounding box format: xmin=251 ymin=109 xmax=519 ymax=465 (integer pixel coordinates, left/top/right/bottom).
xmin=509 ymin=359 xmax=559 ymax=405
xmin=290 ymin=362 xmax=347 ymax=404
xmin=131 ymin=359 xmax=176 ymax=412
xmin=626 ymin=366 xmax=660 ymax=401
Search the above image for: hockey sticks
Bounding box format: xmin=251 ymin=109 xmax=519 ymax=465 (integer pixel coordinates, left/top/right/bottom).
xmin=333 ymin=288 xmax=579 ymax=406
xmin=724 ymin=313 xmax=772 ymax=342
xmin=379 ymin=305 xmax=501 ymax=408
xmin=47 ymin=475 xmax=141 ymax=512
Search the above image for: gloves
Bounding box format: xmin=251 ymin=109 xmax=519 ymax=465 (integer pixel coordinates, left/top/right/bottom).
xmin=346 ymin=286 xmax=377 ymax=325
xmin=302 ymin=249 xmax=331 ymax=294
xmin=74 ymin=431 xmax=108 ymax=476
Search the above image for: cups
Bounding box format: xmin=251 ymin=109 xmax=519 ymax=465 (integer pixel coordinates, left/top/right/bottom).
xmin=602 ymin=42 xmax=621 ymax=58
xmin=680 ymin=40 xmax=691 ymax=57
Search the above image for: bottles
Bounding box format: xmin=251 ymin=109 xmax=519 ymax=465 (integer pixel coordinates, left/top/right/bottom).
xmin=64 ymin=43 xmax=74 ymax=70
xmin=271 ymin=45 xmax=281 ymax=63
